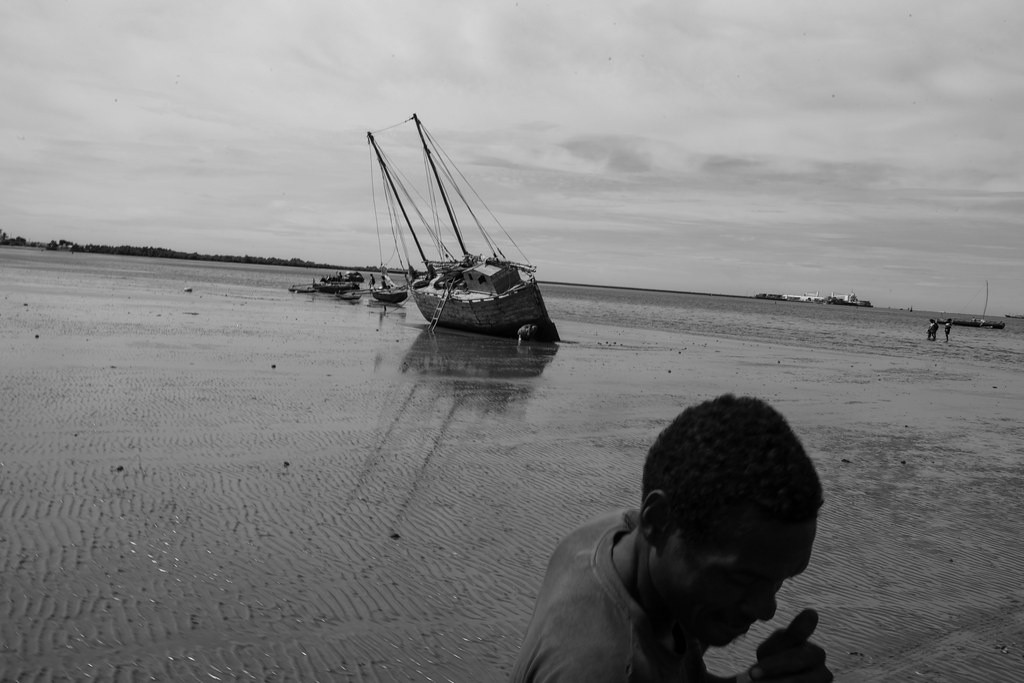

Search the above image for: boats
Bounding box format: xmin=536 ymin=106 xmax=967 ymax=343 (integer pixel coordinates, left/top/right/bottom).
xmin=937 ymin=277 xmax=1005 ymax=328
xmin=756 ymin=286 xmax=873 ymax=307
xmin=290 ymin=271 xmax=407 ymax=302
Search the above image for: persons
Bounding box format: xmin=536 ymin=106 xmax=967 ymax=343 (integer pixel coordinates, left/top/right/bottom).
xmin=319 ymin=269 xmax=392 ymax=291
xmin=945 ymin=318 xmax=952 ymax=340
xmin=507 ymin=394 xmax=834 ymax=683
xmin=929 ymin=319 xmax=939 ymax=340
xmin=517 ymin=323 xmax=537 ymax=344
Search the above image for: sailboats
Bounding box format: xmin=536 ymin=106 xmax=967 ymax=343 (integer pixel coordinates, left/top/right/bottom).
xmin=366 ymin=112 xmax=562 ymax=343
